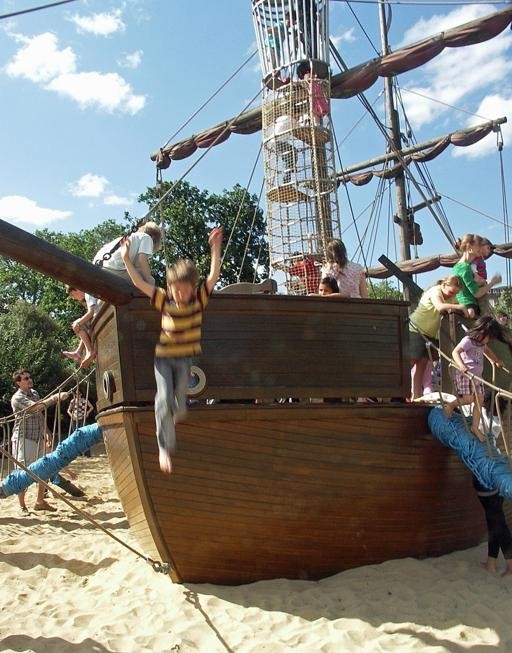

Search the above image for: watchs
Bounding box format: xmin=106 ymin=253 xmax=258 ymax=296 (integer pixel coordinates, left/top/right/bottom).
xmin=76 ymin=319 xmax=82 ymax=327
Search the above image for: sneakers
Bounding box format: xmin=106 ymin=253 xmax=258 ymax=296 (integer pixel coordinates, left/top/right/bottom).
xmin=34 ymin=503 xmax=59 ymax=512
xmin=17 ymin=505 xmax=32 ymax=517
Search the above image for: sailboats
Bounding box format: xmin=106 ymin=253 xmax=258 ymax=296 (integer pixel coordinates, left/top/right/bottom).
xmin=0 ymin=0 xmax=512 ymax=584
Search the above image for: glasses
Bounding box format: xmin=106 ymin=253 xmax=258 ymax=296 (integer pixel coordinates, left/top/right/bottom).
xmin=21 ymin=375 xmax=33 ymax=381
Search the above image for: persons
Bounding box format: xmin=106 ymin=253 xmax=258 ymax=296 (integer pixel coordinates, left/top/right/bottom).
xmin=119 ymin=225 xmax=223 ymax=471
xmin=444 ymin=314 xmax=506 ymax=442
xmin=470 ymin=237 xmax=492 ymax=317
xmin=411 ymin=386 xmax=512 ymax=575
xmin=264 ymin=0 xmax=368 ymax=298
xmin=453 ymin=233 xmax=503 ymax=314
xmin=67 ymin=387 xmax=95 ymax=457
xmin=92 ymin=220 xmax=162 ymax=285
xmin=9 ymin=365 xmax=71 ymax=517
xmin=380 ymin=274 xmax=469 ymax=402
xmin=60 ymin=281 xmax=106 ymax=368
